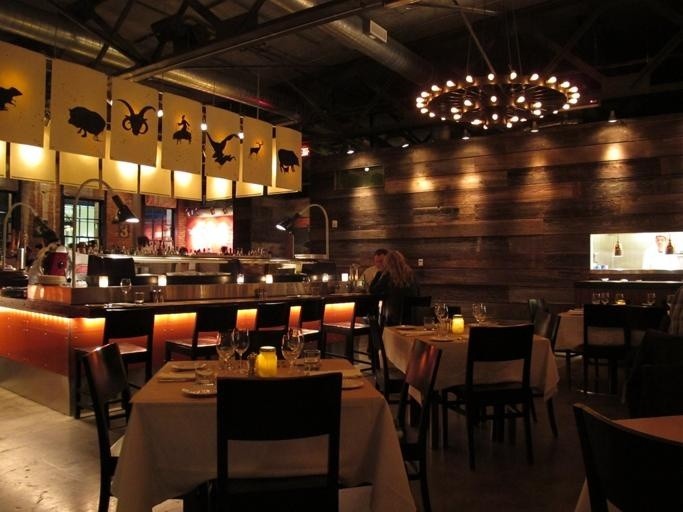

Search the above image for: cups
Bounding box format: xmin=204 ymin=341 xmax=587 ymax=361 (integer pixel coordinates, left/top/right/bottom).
xmin=135 ymin=292 xmax=144 ymax=304
xmin=303 ymin=350 xmax=321 ymax=372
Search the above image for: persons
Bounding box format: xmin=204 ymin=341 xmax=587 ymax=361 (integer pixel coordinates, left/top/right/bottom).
xmin=643 ymin=234 xmax=678 ymax=271
xmin=368 ymin=251 xmax=423 ymax=370
xmin=221 ymin=247 xmax=229 ymax=255
xmin=29 ymin=230 xmax=69 ymax=284
xmin=359 ymin=249 xmax=390 ymax=358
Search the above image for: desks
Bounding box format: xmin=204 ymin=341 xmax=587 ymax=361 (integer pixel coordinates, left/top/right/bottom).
xmin=574 ymin=280 xmax=683 ymax=315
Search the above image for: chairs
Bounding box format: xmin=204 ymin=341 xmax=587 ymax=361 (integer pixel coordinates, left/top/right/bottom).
xmin=365 ymin=319 xmax=410 ymax=403
xmin=393 ymin=338 xmax=441 ymax=509
xmin=573 ymin=401 xmax=683 ymax=510
xmin=321 ymin=297 xmax=380 ymax=373
xmin=528 ymin=297 xmax=546 ymax=323
xmin=165 ymin=307 xmax=238 ymax=360
xmin=397 ymin=295 xmax=460 ymax=325
xmin=245 ymin=302 xmax=288 ymax=360
xmin=82 ymin=344 xmax=141 ymax=512
xmin=441 ymin=325 xmax=534 ymax=469
xmin=76 ymin=310 xmax=152 ymax=422
xmin=583 ymin=305 xmax=630 ymax=400
xmin=531 ymin=309 xmax=561 ymax=437
xmin=184 ymin=373 xmax=339 ymax=512
xmin=288 ymin=303 xmax=321 ymax=353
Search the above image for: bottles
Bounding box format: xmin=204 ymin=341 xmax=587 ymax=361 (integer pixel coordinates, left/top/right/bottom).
xmin=257 ymin=346 xmax=277 ymax=378
xmin=188 ymin=247 xmax=267 ymax=256
xmin=102 ymin=241 xmax=180 ymax=257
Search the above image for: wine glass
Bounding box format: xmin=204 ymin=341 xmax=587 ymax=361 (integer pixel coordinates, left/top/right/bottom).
xmin=231 ymin=328 xmax=251 ymax=375
xmin=120 ymin=279 xmax=132 ymax=304
xmin=591 ymin=292 xmax=675 ymax=308
xmin=216 ymin=330 xmax=236 ymax=372
xmin=281 ymin=328 xmax=304 ymax=376
xmin=433 ymin=302 xmax=486 ymax=336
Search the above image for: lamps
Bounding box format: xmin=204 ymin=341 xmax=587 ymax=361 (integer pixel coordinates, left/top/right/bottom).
xmin=415 ymin=0 xmax=581 ymax=130
xmin=72 ymin=179 xmax=140 ymax=288
xmin=4 ymin=202 xmax=60 ymax=264
xmin=666 ymin=233 xmax=674 ymax=254
xmin=615 ymin=233 xmax=621 ymax=256
xmin=275 ymin=203 xmax=330 ymax=262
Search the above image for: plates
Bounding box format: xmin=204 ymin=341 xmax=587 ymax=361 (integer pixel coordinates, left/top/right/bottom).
xmin=335 ymin=369 xmax=362 ymax=378
xmin=568 ymin=312 xmax=584 ymax=316
xmin=171 ymin=363 xmax=207 ymax=372
xmin=341 ymin=379 xmax=364 ymax=390
xmin=182 ymin=384 xmax=217 ymax=398
